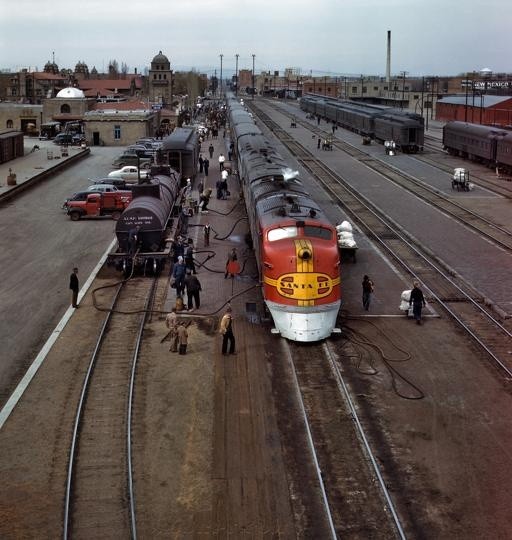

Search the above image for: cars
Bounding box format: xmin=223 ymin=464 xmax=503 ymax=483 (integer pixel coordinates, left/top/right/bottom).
xmin=61 ymin=137 xmax=163 ymax=221
xmin=54 ymin=132 xmax=82 ymax=145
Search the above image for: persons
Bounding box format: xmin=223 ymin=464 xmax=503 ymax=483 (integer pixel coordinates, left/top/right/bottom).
xmin=184 ymin=268 xmax=202 ymax=310
xmin=219 ymin=307 xmax=238 ymax=356
xmin=223 ymin=247 xmax=238 ymax=279
xmin=185 ymin=244 xmax=199 ymax=274
xmin=70 ymin=267 xmax=81 ymax=309
xmin=409 ymin=280 xmax=426 ymax=325
xmin=362 ymin=275 xmax=376 ymax=312
xmin=173 ymin=141 xmax=234 ymax=262
xmin=153 ymin=103 xmax=229 ymax=143
xmin=164 ymin=307 xmax=179 ymax=352
xmin=174 ymin=320 xmax=188 ymax=355
xmin=172 ymin=255 xmax=187 ymax=298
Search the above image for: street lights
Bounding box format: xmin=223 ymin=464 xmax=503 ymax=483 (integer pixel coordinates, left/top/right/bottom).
xmin=40 ymin=107 xmax=44 ymax=123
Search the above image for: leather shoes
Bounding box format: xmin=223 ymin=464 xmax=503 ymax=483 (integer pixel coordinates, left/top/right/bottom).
xmin=220 ymin=351 xmax=237 ymax=357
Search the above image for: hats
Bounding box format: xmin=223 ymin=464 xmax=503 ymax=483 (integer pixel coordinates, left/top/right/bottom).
xmin=226 ymin=308 xmax=233 ymax=313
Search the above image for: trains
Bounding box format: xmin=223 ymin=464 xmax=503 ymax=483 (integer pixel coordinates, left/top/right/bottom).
xmin=107 ymin=124 xmax=201 ymax=276
xmin=298 ymin=91 xmax=426 ymax=155
xmin=222 ymin=91 xmax=345 ymax=344
xmin=442 ymin=120 xmax=512 ymax=173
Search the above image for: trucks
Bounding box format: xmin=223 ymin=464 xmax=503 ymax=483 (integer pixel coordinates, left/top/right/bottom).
xmin=64 ymin=124 xmax=83 ymax=138
xmin=39 ymin=121 xmax=61 ymax=142
